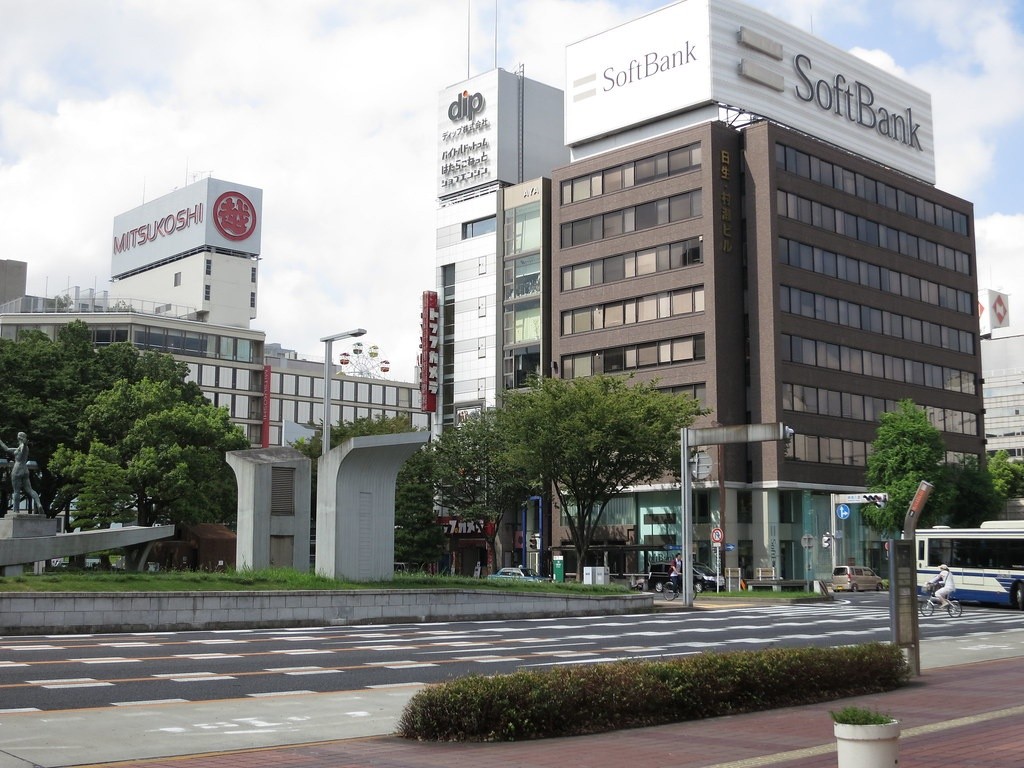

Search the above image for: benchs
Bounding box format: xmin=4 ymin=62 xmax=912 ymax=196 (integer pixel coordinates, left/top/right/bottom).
xmin=746 ymin=580 xmax=824 ymax=592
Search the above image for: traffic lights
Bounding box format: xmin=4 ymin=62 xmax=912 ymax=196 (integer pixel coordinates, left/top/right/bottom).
xmin=873 ymin=502 xmax=884 ymax=509
xmin=863 ymin=494 xmax=883 ymax=501
xmin=529 ymin=539 xmax=536 ymax=549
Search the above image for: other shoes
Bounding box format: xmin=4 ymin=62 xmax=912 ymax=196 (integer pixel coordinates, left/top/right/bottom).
xmin=940 ymin=602 xmax=948 ymax=608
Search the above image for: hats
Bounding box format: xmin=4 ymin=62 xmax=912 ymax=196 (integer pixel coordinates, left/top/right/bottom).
xmin=938 ymin=563 xmax=949 ymax=569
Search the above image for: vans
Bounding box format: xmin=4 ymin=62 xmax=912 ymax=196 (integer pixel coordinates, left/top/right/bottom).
xmin=831 ymin=566 xmax=884 ymax=592
xmin=648 ymin=562 xmax=726 ymax=593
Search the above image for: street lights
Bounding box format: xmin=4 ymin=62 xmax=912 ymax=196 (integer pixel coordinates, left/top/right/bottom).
xmin=530 ymin=496 xmax=543 ymax=576
xmin=320 ymin=329 xmax=367 ymax=457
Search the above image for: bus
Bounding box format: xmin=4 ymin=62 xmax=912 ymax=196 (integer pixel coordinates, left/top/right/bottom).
xmin=900 ymin=521 xmax=1024 ymax=611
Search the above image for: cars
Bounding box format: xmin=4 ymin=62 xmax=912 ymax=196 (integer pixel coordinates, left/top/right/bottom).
xmin=487 ymin=567 xmax=552 ymax=583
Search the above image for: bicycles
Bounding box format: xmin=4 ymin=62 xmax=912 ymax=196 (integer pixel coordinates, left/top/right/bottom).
xmin=663 ymin=573 xmax=697 ymax=601
xmin=920 ymin=582 xmax=962 ymax=618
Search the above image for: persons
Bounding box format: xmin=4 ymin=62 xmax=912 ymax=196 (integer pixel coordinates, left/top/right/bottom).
xmin=667 ymin=552 xmax=683 ymax=598
xmin=7 ymin=432 xmax=45 ymax=515
xmin=925 ymin=564 xmax=956 ymax=609
